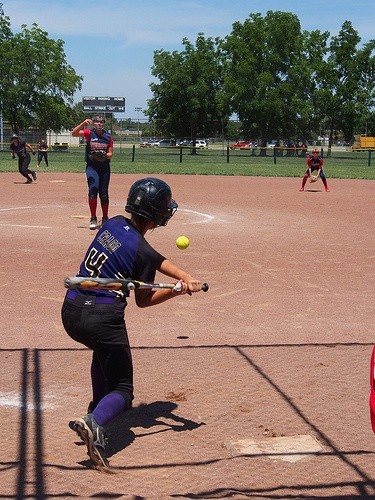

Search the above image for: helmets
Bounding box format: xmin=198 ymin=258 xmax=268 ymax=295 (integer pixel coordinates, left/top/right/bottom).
xmin=312 ymin=149 xmax=319 ymax=157
xmin=11 ymin=134 xmax=19 ymax=142
xmin=125 ymin=178 xmax=178 ymax=227
xmin=92 ymin=114 xmax=106 ymax=130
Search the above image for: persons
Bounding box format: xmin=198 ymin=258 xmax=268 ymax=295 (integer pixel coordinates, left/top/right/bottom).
xmin=275 ymin=139 xmax=306 ymax=156
xmin=10 ymin=133 xmax=36 ymax=183
xmin=299 ymin=148 xmax=329 ymax=192
xmin=60 ymin=177 xmax=203 ymax=471
xmin=37 ymin=140 xmax=48 ymax=167
xmin=72 ymin=114 xmax=113 ymax=229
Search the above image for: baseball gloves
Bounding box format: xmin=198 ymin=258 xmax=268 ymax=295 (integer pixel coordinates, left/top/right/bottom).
xmin=89 ymin=149 xmax=108 ymax=164
xmin=310 ymin=176 xmax=318 ymax=182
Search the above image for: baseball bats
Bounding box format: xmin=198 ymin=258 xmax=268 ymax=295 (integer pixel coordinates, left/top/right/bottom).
xmin=63 ymin=275 xmax=209 ymax=294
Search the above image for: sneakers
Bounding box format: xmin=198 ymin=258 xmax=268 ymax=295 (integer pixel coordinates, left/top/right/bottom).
xmin=69 ymin=414 xmax=109 ymax=468
xmin=100 ymin=216 xmax=109 ymax=228
xmin=90 ymin=216 xmax=98 ymax=229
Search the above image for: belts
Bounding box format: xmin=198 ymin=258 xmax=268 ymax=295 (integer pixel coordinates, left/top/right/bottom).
xmin=66 ymin=289 xmax=115 ymax=304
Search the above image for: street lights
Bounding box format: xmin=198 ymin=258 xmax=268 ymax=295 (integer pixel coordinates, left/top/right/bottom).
xmin=135 ymin=107 xmax=142 ymax=136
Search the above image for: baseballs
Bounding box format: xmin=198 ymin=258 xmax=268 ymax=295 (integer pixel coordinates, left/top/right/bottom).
xmin=175 ymin=236 xmax=190 ymax=250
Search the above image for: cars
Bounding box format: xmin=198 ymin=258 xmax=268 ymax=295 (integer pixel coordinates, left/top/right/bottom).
xmin=140 ymin=138 xmax=176 ymax=146
xmin=230 ymin=141 xmax=277 ymax=149
xmin=177 ymin=138 xmax=207 ymax=147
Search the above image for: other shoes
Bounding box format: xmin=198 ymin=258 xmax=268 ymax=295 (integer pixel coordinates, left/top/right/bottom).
xmin=24 ymin=178 xmax=32 ymax=184
xmin=33 ymin=171 xmax=36 ymax=180
xmin=299 ymin=188 xmax=304 ymax=191
xmin=325 ymin=188 xmax=330 ymax=192
